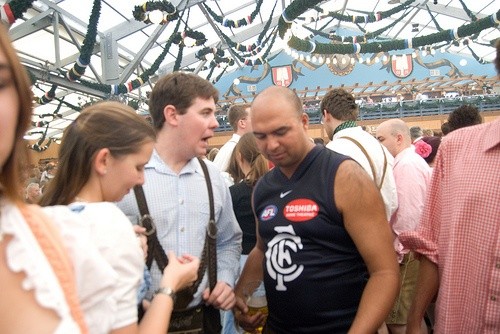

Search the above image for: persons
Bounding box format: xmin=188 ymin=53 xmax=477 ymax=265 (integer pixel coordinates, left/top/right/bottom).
xmin=384 ymin=118 xmax=431 ymax=334
xmin=409 ymin=104 xmax=482 ymax=169
xmin=116 ymin=68 xmax=243 ymax=334
xmin=0 ymin=19 xmax=116 ymax=334
xmin=233 ymin=85 xmax=401 ymax=334
xmin=400 ymin=39 xmax=500 ymax=334
xmin=318 ymin=88 xmax=399 ymax=222
xmin=43 ymin=96 xmax=201 ymax=334
xmin=29 ymin=105 xmax=270 ymax=207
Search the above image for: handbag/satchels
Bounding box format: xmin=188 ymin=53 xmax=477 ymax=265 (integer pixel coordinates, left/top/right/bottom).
xmin=138 ymin=296 xmax=224 ymax=334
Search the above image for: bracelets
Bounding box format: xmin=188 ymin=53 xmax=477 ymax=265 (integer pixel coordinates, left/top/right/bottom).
xmin=155 ymin=286 xmax=175 ymax=297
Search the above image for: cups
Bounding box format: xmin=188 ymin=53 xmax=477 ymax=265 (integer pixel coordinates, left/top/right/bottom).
xmin=243 ymin=281 xmax=267 ymax=334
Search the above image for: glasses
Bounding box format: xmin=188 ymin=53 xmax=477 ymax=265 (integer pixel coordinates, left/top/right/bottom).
xmin=318 ymin=114 xmax=324 ymax=125
